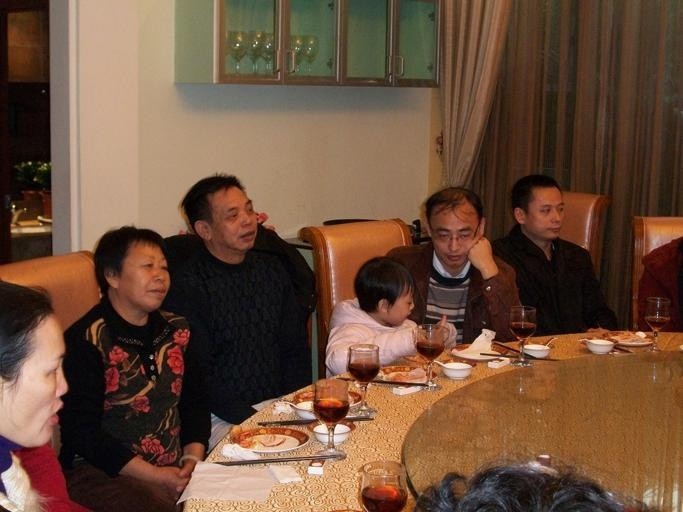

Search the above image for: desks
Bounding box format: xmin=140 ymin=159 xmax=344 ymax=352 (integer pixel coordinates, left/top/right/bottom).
xmin=283 ymin=225 xmax=431 ymax=383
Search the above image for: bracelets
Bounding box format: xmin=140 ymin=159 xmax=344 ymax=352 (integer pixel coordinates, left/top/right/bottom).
xmin=178 ymin=455 xmax=198 ymax=467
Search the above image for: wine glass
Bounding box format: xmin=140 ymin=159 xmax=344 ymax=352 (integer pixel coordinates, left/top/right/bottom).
xmin=224 ymin=29 xmax=317 ymax=77
xmin=412 ymin=323 xmax=446 ymax=391
xmin=508 ymin=304 xmax=536 ymax=368
xmin=643 ymin=296 xmax=671 ymax=351
xmin=348 ymin=343 xmax=379 ymax=421
xmin=313 ymin=376 xmax=349 ymax=461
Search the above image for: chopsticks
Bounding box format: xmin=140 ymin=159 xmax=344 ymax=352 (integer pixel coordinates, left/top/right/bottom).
xmin=209 ymin=454 xmax=337 ymax=467
xmin=336 ymin=377 xmax=429 ymax=387
xmin=257 ymin=416 xmax=375 ymax=427
xmin=477 ymin=342 xmax=560 ymax=363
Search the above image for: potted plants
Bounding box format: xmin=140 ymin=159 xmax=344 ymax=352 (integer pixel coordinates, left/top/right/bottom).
xmin=13 ymin=160 xmax=41 ymax=211
xmin=33 ymin=160 xmax=53 ymax=221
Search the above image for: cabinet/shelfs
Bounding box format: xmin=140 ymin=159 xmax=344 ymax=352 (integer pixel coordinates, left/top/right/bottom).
xmin=171 ymin=0 xmax=442 ymax=87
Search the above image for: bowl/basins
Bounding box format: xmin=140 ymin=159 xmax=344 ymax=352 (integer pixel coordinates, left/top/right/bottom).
xmin=440 ymin=362 xmax=472 ymax=381
xmin=521 ymin=345 xmax=550 ymax=360
xmin=294 ymin=401 xmax=322 ymax=419
xmin=312 ymin=422 xmax=350 ymax=446
xmin=582 ymin=339 xmax=614 ymax=355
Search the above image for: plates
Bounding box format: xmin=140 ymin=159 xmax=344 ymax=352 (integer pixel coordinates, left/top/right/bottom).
xmin=373 ymin=365 xmax=437 ymax=384
xmin=448 ymin=342 xmax=509 ymax=361
xmin=599 ymin=331 xmax=657 ymax=347
xmin=234 ymin=427 xmax=311 ymax=455
xmin=291 ymin=389 xmax=363 ymax=409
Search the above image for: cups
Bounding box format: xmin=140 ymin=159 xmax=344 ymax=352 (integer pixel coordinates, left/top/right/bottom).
xmin=359 ymin=460 xmax=408 ymax=512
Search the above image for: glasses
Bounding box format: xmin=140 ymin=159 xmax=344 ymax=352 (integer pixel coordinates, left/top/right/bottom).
xmin=429 ymin=223 xmax=480 ymax=242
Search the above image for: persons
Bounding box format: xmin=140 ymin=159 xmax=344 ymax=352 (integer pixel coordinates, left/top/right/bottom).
xmin=386 ymin=187 xmax=528 ymax=343
xmin=325 ymin=256 xmax=458 ymax=378
xmin=413 ymin=459 xmax=651 ymax=512
xmin=490 ymin=174 xmax=618 ymax=337
xmin=637 ymin=236 xmax=683 ymax=332
xmin=57 ymin=226 xmax=212 ymax=512
xmin=157 ymin=176 xmax=317 ymax=456
xmin=1 ymin=280 xmax=91 ymax=512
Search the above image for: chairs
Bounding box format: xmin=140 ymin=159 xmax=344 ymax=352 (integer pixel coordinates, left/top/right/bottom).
xmin=629 ymin=214 xmax=683 ymax=332
xmin=0 ymin=251 xmax=100 ymax=334
xmin=557 ymin=189 xmax=611 ymax=283
xmin=298 ymin=216 xmax=413 ymax=380
